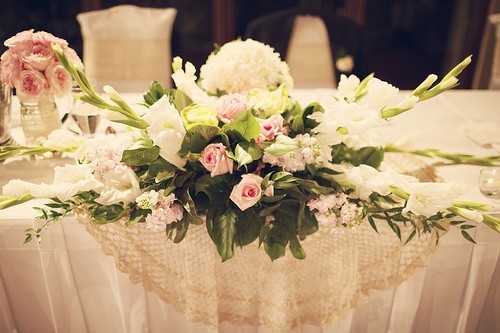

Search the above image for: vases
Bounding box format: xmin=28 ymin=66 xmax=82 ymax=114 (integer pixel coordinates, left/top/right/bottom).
xmin=16 ymin=90 xmax=61 ymax=136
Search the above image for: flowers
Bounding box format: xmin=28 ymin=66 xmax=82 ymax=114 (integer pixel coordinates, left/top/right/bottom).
xmin=0 ymin=38 xmax=500 ymax=262
xmin=0 ymin=29 xmax=84 ymax=103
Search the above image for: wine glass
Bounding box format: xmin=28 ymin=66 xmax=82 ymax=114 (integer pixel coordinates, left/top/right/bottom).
xmin=459 ymin=0 xmax=500 ymax=215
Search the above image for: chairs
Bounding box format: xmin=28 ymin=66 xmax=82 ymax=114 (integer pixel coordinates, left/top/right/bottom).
xmin=248 ymin=10 xmax=374 ymax=93
xmin=77 ymin=4 xmax=178 ymax=95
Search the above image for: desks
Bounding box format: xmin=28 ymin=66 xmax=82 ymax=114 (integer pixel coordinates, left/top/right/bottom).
xmin=0 ymin=90 xmax=500 ymax=333
